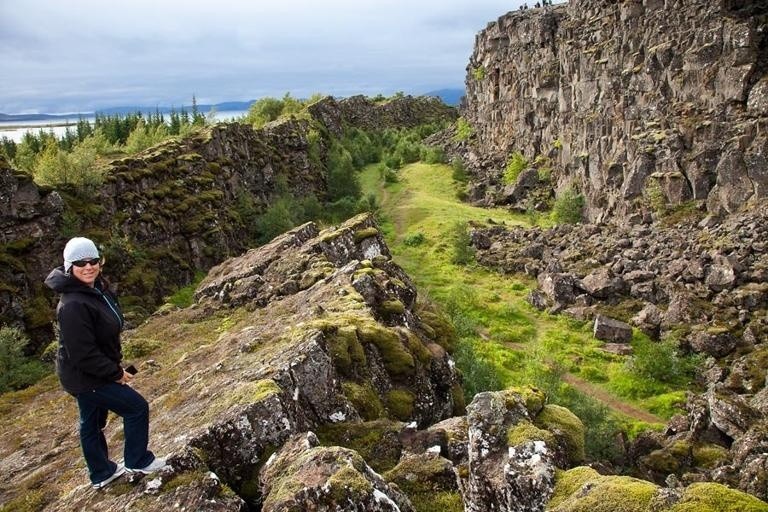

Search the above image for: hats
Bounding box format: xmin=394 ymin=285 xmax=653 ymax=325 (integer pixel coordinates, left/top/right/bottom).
xmin=63 ymin=236 xmax=101 ymax=277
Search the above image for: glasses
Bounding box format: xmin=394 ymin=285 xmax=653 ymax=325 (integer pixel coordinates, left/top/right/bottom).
xmin=72 ymin=258 xmax=101 ymax=267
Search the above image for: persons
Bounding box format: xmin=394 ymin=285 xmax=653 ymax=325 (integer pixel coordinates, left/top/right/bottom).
xmin=43 ymin=236 xmax=166 ymax=490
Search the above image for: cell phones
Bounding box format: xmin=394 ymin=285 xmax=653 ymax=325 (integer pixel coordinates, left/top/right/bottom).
xmin=124 ymin=365 xmax=138 ymax=376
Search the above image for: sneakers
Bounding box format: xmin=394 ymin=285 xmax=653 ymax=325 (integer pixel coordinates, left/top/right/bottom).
xmin=92 ymin=462 xmax=126 ymax=490
xmin=124 ymin=457 xmax=167 ymax=475
